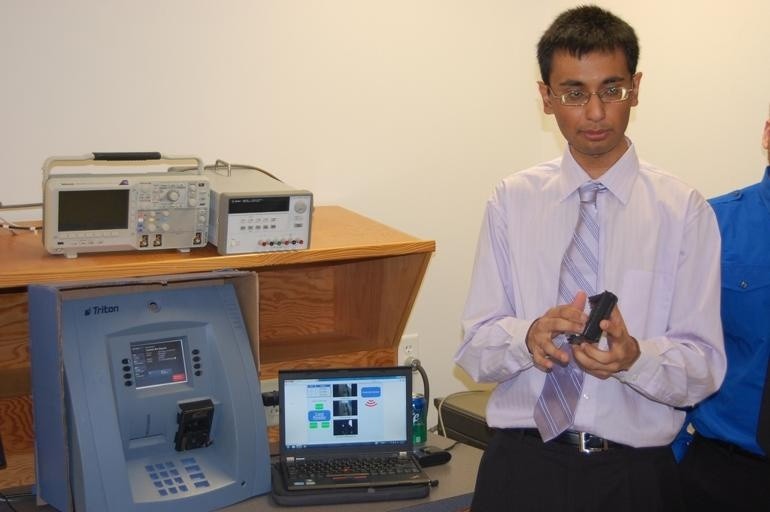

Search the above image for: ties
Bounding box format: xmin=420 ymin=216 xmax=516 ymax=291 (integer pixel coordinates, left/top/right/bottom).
xmin=532 ymin=182 xmax=602 ymax=445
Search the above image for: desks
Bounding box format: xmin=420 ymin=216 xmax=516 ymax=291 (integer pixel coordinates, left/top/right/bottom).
xmin=0 ymin=429 xmax=484 ymax=512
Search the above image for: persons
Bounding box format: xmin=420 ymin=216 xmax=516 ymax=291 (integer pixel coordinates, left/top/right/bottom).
xmin=673 ymin=126 xmax=770 ymax=512
xmin=454 ymin=5 xmax=727 ymax=511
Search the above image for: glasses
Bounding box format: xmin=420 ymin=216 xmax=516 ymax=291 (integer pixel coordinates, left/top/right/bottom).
xmin=548 ymin=78 xmax=634 ymax=107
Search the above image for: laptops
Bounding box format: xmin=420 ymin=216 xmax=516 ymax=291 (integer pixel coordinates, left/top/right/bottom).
xmin=278 ymin=365 xmax=431 ymax=492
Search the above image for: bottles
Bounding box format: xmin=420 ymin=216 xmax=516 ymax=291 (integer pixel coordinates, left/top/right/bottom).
xmin=413 ymin=393 xmax=428 ymax=449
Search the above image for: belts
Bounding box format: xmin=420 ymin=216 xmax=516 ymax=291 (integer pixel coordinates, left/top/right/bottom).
xmin=496 ymin=421 xmax=659 ymax=457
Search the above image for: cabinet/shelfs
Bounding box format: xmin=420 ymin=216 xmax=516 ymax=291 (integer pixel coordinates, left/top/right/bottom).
xmin=433 ymin=392 xmax=486 ymax=450
